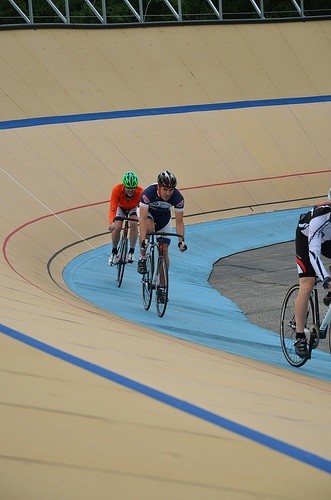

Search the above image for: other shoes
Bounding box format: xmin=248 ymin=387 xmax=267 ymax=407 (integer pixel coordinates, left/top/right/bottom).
xmin=158 ymin=290 xmax=169 ymax=303
xmin=138 ymin=258 xmax=147 ymax=274
xmin=293 ymin=337 xmax=309 ymax=357
xmin=109 ymin=254 xmax=117 ymax=266
xmin=128 ymin=252 xmax=135 ymax=262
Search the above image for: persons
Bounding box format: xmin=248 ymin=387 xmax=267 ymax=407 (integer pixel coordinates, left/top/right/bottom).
xmin=137 ymin=170 xmax=187 ymax=294
xmin=294 ymin=187 xmax=331 ymax=358
xmin=108 ymin=171 xmax=145 ymax=266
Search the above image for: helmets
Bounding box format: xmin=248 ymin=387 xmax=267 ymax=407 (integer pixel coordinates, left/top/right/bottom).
xmin=157 ymin=171 xmax=176 ymax=187
xmin=122 ymin=171 xmax=137 ymax=188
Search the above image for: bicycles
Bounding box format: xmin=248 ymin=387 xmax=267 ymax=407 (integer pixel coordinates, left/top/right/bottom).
xmin=137 ymin=230 xmax=186 ymax=318
xmin=109 ymin=210 xmax=139 ymax=288
xmin=280 ymin=270 xmax=331 ymax=368
